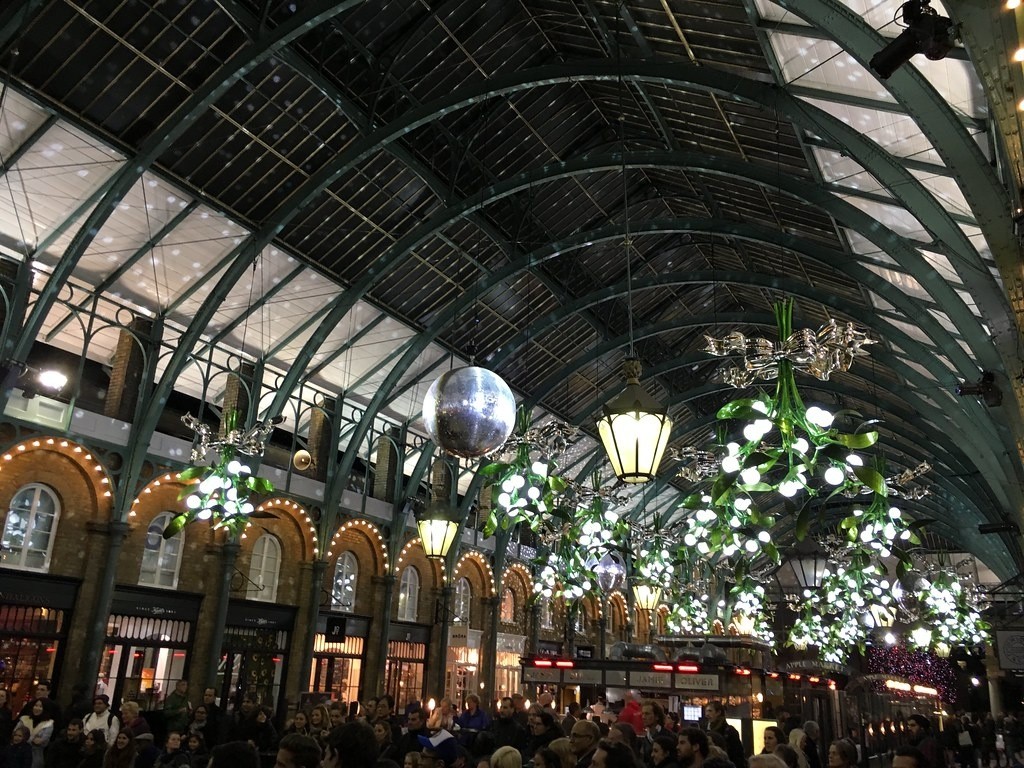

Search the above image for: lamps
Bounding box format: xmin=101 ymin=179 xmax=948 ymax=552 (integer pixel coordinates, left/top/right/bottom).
xmin=155 ymin=254 xmax=290 ymax=544
xmin=587 ymin=54 xmax=676 ymax=487
xmin=412 ymin=247 xmax=464 ymax=559
xmin=476 ymin=228 xmax=567 ymax=537
xmin=705 ymin=90 xmax=895 ymax=506
xmin=519 ymin=451 xmax=997 ymax=665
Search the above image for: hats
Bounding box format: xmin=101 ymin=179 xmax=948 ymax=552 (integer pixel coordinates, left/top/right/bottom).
xmin=418 ymin=729 xmax=459 ymax=759
xmin=92 ymin=694 xmax=111 ymax=707
xmin=908 ymin=714 xmax=932 ymax=731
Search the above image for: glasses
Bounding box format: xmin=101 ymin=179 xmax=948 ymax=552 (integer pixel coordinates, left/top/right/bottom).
xmin=94 ymin=702 xmax=104 ymax=705
xmin=567 ymin=732 xmax=592 ymax=739
xmin=907 ymin=723 xmax=917 ymax=727
xmin=420 ymin=751 xmax=435 ymax=759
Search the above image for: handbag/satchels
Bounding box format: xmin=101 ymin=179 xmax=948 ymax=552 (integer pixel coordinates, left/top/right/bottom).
xmin=957 ymin=725 xmax=973 ymax=744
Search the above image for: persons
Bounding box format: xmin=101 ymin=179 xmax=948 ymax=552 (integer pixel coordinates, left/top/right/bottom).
xmin=0 ymin=679 xmax=1024 ymax=768
xmin=94 ymin=672 xmax=107 ymax=695
